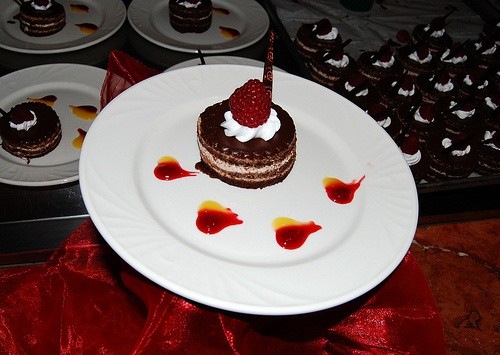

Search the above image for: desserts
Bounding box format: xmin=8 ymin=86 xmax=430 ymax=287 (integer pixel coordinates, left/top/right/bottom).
xmin=168 ymin=0 xmax=212 ymax=34
xmin=294 ymin=10 xmax=500 ymax=182
xmin=0 ymin=101 xmax=61 ymax=159
xmin=197 ymin=30 xmax=297 ymax=189
xmin=19 ymin=0 xmax=66 ymax=37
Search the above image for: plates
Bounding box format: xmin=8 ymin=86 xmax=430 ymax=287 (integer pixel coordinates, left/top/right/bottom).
xmin=0 ymin=0 xmax=126 ymax=54
xmin=0 ymin=62 xmax=130 ymax=187
xmin=163 ymin=56 xmax=288 ymax=73
xmin=78 ymin=64 xmax=418 ymax=316
xmin=128 ymin=0 xmax=269 ymax=53
